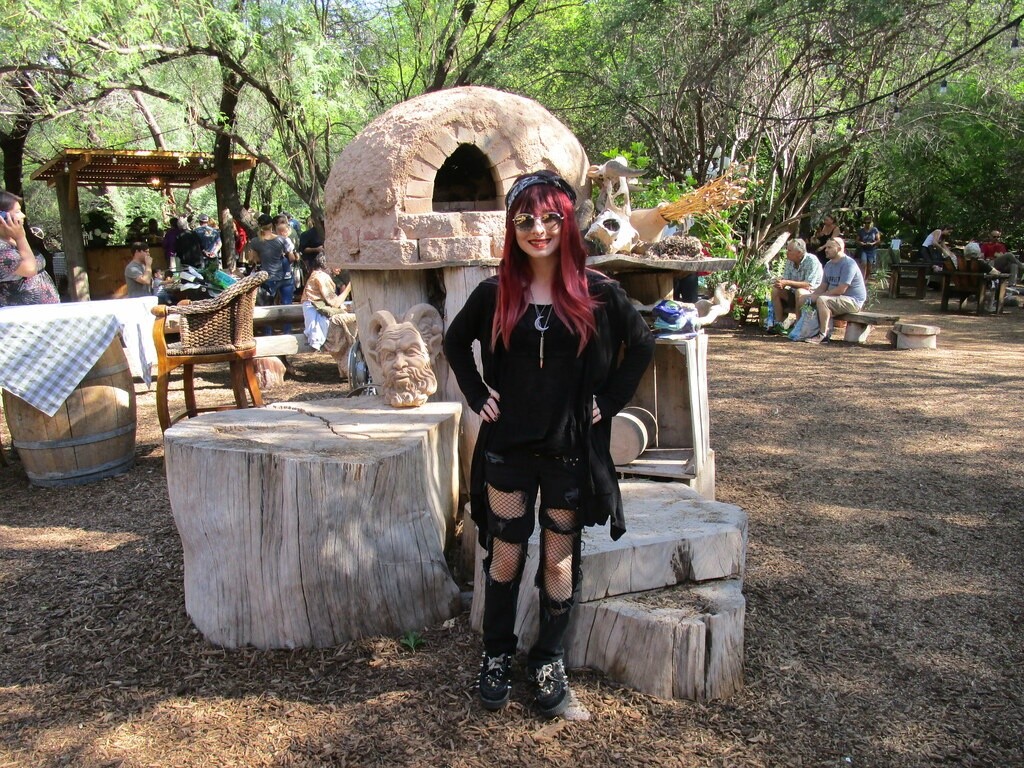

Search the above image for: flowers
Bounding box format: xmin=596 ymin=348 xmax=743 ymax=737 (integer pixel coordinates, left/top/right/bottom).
xmin=700 ymin=240 xmax=769 ymax=319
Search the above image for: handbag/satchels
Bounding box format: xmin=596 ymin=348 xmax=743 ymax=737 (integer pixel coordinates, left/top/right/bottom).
xmin=787 ymin=308 xmax=833 ymax=342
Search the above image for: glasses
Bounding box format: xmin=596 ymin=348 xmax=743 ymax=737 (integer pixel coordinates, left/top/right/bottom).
xmin=791 ymin=238 xmax=801 ymax=248
xmin=864 ymin=221 xmax=870 ymax=224
xmin=512 ymin=212 xmax=564 ymax=231
xmin=991 ymin=235 xmax=1000 ymax=238
xmin=829 ymin=238 xmax=840 ymax=246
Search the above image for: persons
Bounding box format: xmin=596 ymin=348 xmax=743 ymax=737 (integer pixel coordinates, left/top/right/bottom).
xmin=375 ymin=324 xmax=438 ymax=407
xmin=767 ymin=238 xmax=824 ymax=334
xmin=300 ymin=253 xmax=351 ymax=351
xmin=922 ymin=225 xmax=953 ymax=284
xmin=246 ymin=212 xmax=321 ymax=335
xmin=159 ymin=213 xmax=247 ymax=273
xmin=151 ymin=268 xmax=176 ymax=305
xmin=124 ymin=242 xmax=155 ymax=299
xmin=795 ymin=237 xmax=866 ymax=345
xmin=125 ymin=216 xmax=164 ymax=245
xmin=815 ymin=215 xmax=840 ymax=265
xmin=855 ymin=215 xmax=881 ymax=278
xmin=0 ymin=188 xmax=61 ymax=306
xmin=441 ymin=171 xmax=653 ymax=716
xmin=963 ymin=229 xmax=1024 ymax=286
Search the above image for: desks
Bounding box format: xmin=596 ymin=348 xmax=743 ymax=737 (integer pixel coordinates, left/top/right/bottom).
xmin=163 ymin=302 xmax=355 ymax=336
xmin=0 ymin=295 xmax=159 ymax=390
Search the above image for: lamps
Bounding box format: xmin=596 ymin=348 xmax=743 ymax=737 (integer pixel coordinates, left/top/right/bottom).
xmin=145 ymin=172 xmax=167 ymax=193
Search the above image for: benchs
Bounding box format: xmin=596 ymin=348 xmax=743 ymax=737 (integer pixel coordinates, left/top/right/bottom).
xmin=783 ymin=306 xmax=901 ymax=343
xmin=251 ymin=333 xmax=327 ymax=390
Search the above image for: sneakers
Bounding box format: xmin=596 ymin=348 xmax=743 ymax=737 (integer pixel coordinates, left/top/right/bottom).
xmin=479 ymin=651 xmax=512 ymax=708
xmin=525 ymin=657 xmax=571 ymax=718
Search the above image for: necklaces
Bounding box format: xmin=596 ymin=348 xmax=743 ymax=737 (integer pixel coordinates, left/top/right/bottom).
xmin=529 ymin=289 xmax=556 ymax=369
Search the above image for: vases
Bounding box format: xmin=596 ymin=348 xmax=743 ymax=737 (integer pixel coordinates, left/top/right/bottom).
xmin=630 ymin=203 xmax=671 ymax=244
xmin=702 ymin=307 xmax=751 ymax=329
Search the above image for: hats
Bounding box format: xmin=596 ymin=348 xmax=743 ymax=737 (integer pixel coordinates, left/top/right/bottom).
xmin=199 ymin=214 xmax=209 ymax=223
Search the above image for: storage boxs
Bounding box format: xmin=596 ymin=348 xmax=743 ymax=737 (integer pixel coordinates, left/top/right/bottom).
xmin=612 ymin=328 xmax=715 ymax=499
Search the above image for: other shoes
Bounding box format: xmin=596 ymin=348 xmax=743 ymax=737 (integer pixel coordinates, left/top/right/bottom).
xmin=804 ymin=331 xmax=828 ymax=343
xmin=764 ymin=322 xmax=784 ymax=337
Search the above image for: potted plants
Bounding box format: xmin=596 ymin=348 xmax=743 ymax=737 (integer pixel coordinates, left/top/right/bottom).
xmin=83 ymin=210 xmax=116 ymax=249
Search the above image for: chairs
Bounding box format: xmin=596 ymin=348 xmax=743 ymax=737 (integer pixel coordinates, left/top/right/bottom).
xmin=887 ymin=240 xmax=930 ymax=299
xmin=151 ymin=269 xmax=265 ymax=477
xmin=939 ymin=249 xmax=1012 ymax=315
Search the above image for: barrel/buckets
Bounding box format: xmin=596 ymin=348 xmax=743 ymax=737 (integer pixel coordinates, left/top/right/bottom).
xmin=2 ymin=333 xmax=137 ymax=488
